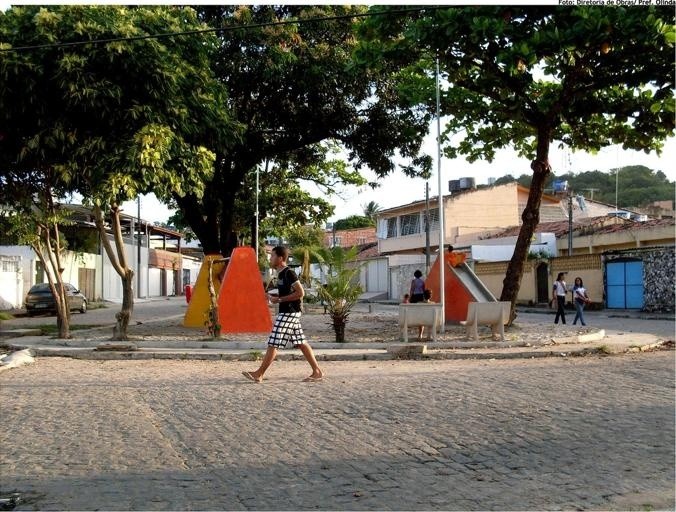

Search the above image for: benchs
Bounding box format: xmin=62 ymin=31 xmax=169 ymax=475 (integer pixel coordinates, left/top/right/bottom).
xmin=398 ymin=300 xmax=512 ymax=344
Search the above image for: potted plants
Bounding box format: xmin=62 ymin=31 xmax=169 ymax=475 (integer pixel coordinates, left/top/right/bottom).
xmin=306 ymin=242 xmax=372 ymax=342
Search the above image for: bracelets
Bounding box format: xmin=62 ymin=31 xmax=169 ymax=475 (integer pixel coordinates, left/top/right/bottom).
xmin=277 ymin=296 xmax=282 ymax=303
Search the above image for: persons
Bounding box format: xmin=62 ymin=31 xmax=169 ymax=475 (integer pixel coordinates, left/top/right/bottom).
xmin=552 ymin=272 xmax=569 ymax=324
xmin=241 ymin=246 xmax=322 ymax=383
xmin=403 ymin=293 xmax=410 ymax=304
xmin=416 ymin=288 xmax=435 ymax=338
xmin=571 ymin=277 xmax=592 ymax=325
xmin=409 ymin=269 xmax=426 ymax=300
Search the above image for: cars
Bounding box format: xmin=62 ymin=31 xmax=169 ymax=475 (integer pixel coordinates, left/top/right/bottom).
xmin=25 ymin=282 xmax=88 ymax=316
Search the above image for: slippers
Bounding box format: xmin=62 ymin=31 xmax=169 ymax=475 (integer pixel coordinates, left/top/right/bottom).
xmin=241 ymin=371 xmax=264 ymax=384
xmin=301 ymin=375 xmax=324 ymax=382
